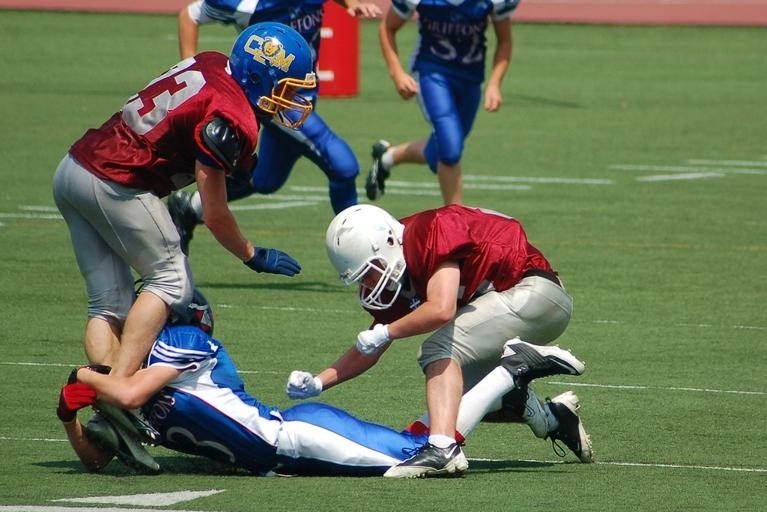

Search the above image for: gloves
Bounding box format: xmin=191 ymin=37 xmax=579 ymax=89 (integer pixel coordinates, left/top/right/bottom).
xmin=355 ymin=324 xmax=391 ymax=357
xmin=56 ymin=382 xmax=96 ymax=421
xmin=285 ymin=369 xmax=324 ymax=400
xmin=245 ymin=245 xmax=302 ymax=278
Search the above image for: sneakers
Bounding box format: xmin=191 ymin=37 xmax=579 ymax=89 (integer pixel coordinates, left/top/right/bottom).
xmin=547 ymin=389 xmax=594 ymax=463
xmin=166 ymin=187 xmax=200 ymax=255
xmin=365 ymin=140 xmax=394 ymax=200
xmin=502 ymin=391 xmax=547 ymax=440
xmin=500 ymin=336 xmax=588 ymax=390
xmin=84 ymin=401 xmax=164 ymax=474
xmin=381 ymin=441 xmax=469 ymax=478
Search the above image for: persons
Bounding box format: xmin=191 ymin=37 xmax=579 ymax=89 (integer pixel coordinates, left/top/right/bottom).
xmin=55 ymin=286 xmax=595 ymax=476
xmin=168 ymin=0 xmax=355 ymax=255
xmin=365 ymin=0 xmax=525 ymax=204
xmin=53 ymin=20 xmax=318 ymax=473
xmin=285 ymin=202 xmax=573 ymax=482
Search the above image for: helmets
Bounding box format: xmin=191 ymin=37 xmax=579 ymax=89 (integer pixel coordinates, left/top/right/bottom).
xmin=326 ymin=202 xmax=408 ymax=312
xmin=229 ymin=17 xmax=317 ymax=131
